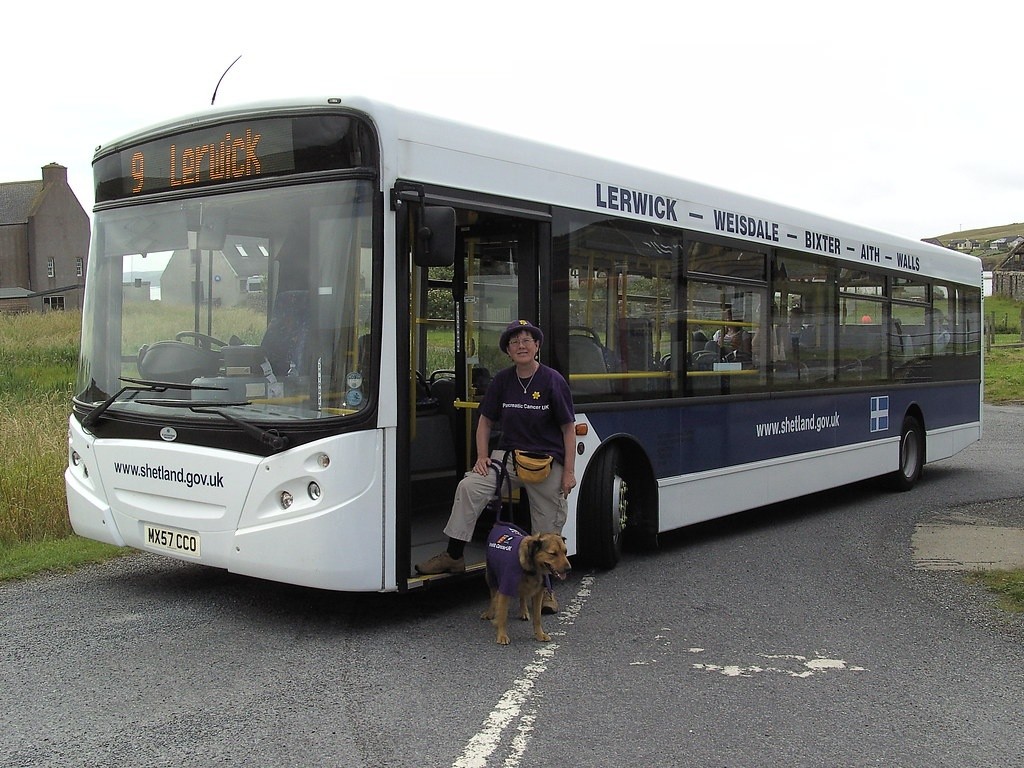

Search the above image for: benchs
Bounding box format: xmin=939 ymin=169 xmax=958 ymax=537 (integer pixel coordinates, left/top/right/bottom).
xmin=749 ymin=360 xmax=809 ymax=386
xmin=802 ymin=356 xmax=863 ymax=381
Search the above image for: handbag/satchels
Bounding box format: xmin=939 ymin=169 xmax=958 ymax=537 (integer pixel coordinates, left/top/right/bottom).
xmin=515 ymin=450 xmax=554 ymax=484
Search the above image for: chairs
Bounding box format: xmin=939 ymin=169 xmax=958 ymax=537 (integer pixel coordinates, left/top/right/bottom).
xmin=430 ymin=369 xmax=456 ymax=445
xmin=260 ymin=290 xmax=316 ymax=377
xmin=569 ymin=325 xmax=615 ymax=396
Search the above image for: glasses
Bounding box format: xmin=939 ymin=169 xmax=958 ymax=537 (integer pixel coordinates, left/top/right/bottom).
xmin=509 ymin=338 xmax=535 ymax=347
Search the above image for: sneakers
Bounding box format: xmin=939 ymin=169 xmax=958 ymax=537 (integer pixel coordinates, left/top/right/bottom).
xmin=415 ymin=550 xmax=465 ymax=573
xmin=541 ymin=587 xmax=558 ymax=615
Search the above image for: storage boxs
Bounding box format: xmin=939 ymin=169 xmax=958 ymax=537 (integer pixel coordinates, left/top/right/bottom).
xmin=220 ymin=345 xmax=265 ymax=375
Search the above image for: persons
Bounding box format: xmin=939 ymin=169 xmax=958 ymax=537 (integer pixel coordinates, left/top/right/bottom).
xmin=713 ymin=309 xmax=752 ymax=356
xmin=416 ymin=320 xmax=576 ymax=614
xmin=914 ymin=307 xmax=951 ymax=353
xmin=784 ymin=307 xmax=817 ymax=352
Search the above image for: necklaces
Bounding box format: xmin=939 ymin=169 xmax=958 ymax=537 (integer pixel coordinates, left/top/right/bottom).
xmin=516 ymin=362 xmax=538 ymax=393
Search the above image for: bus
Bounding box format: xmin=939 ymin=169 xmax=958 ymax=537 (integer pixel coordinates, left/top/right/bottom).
xmin=53 ymin=95 xmax=987 ymax=601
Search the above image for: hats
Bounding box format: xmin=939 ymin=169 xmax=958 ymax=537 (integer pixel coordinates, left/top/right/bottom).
xmin=499 ymin=320 xmax=543 ymax=355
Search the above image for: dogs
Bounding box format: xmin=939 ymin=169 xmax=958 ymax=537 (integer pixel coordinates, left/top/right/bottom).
xmin=480 ymin=522 xmax=571 ymax=645
